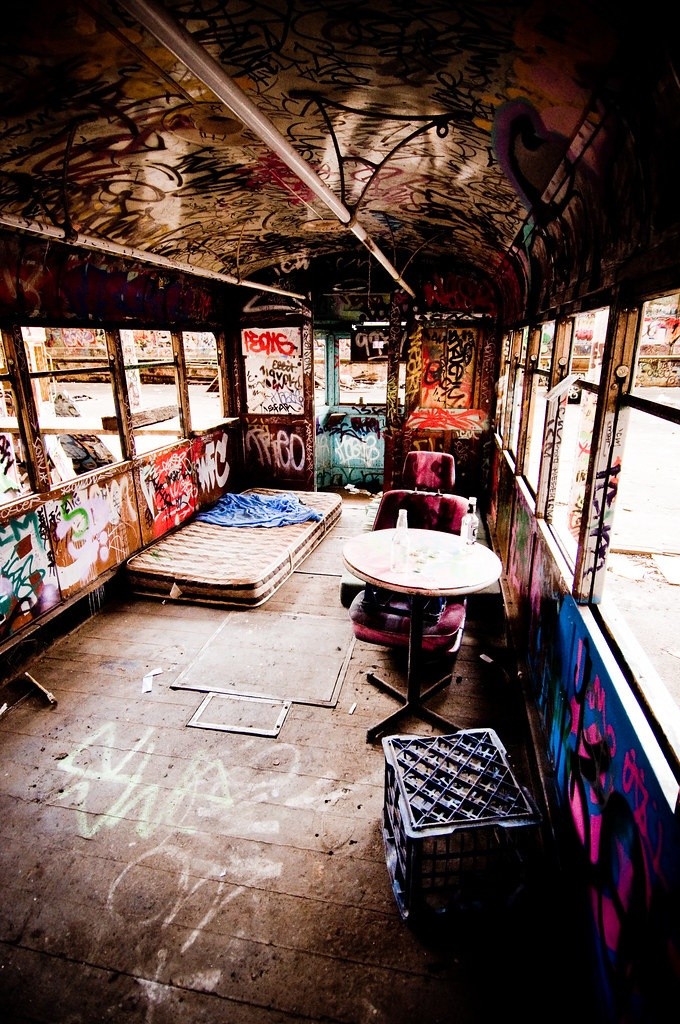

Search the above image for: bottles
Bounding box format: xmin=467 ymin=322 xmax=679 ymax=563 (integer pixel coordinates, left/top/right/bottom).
xmin=390 ymin=509 xmax=409 ymax=573
xmin=461 ymin=497 xmax=479 ymax=553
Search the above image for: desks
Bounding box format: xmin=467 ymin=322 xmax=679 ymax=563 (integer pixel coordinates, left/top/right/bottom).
xmin=342 ymin=526 xmax=501 ymax=743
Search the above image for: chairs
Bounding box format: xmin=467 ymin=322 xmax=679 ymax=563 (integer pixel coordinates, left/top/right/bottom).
xmin=348 ymin=488 xmax=471 ymax=655
xmin=385 ymin=450 xmax=455 ymax=494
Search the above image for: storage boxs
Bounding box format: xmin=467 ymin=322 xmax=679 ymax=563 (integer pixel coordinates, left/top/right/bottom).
xmin=383 ymin=728 xmax=544 ymax=925
